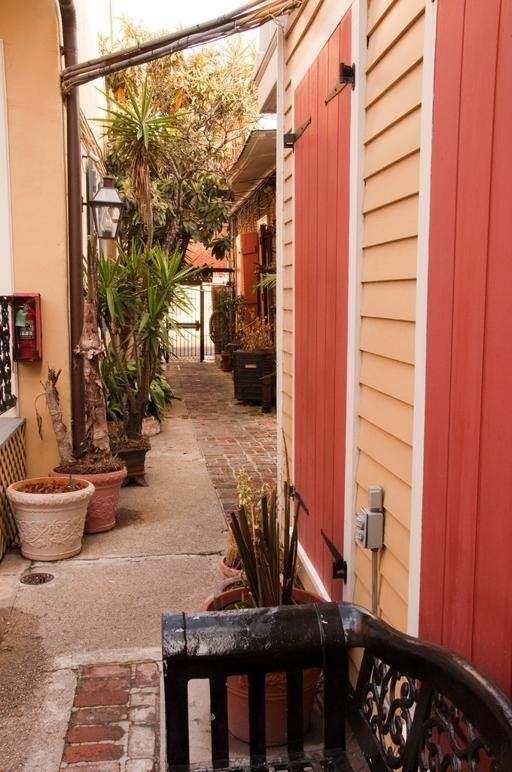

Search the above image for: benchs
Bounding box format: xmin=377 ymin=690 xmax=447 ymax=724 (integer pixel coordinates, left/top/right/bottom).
xmin=160 ymin=602 xmax=511 ymax=772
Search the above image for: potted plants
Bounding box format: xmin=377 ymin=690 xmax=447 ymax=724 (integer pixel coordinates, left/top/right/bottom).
xmin=203 ymin=468 xmax=330 ymax=747
xmin=215 ymin=263 xmax=276 ymax=414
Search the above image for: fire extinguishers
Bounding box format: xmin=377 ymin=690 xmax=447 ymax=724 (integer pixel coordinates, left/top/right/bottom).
xmin=15 ymin=298 xmax=38 ymax=360
